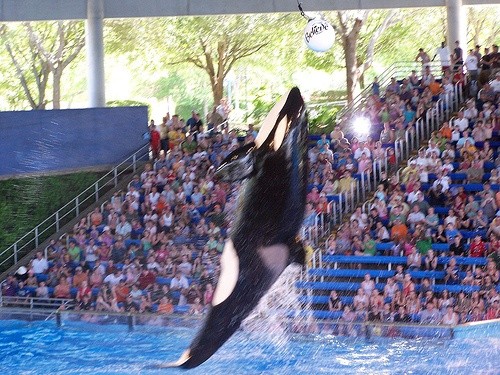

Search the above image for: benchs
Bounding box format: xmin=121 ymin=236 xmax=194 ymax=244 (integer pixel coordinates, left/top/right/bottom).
xmin=1 ymin=91 xmax=500 ymax=323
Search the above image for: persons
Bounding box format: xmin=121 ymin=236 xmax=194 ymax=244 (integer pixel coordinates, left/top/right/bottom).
xmin=271 ymin=39 xmax=500 ymax=341
xmin=0 ymin=100 xmax=261 ymax=328
